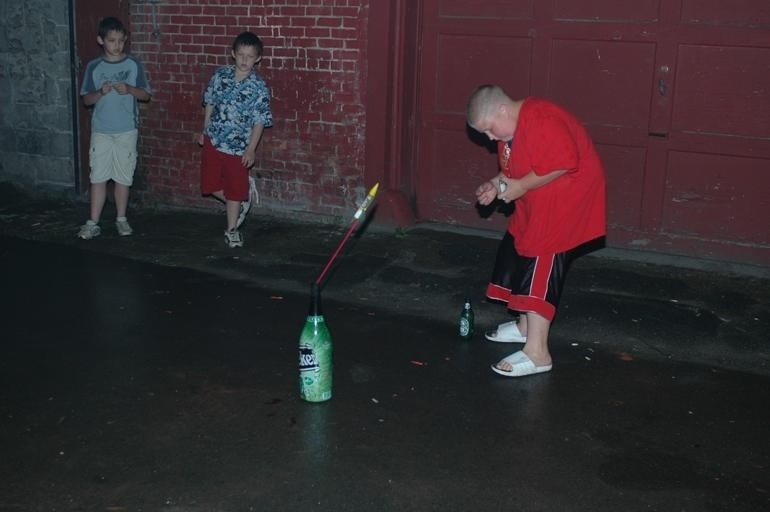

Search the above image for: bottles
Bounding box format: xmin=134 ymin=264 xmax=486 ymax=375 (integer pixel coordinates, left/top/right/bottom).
xmin=458 ymin=297 xmax=475 ymax=340
xmin=297 ymin=283 xmax=333 ymax=403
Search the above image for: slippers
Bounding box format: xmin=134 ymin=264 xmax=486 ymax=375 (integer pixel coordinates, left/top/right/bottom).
xmin=485 ymin=320 xmax=527 ymax=343
xmin=490 ymin=350 xmax=553 ymax=377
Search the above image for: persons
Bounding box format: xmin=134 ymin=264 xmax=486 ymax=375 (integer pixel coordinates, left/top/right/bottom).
xmin=77 ymin=17 xmax=153 ymax=240
xmin=198 ymin=31 xmax=273 ymax=249
xmin=466 ymin=84 xmax=608 ymax=377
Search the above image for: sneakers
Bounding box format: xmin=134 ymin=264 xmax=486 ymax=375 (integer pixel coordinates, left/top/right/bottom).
xmin=115 ymin=217 xmax=133 ymax=236
xmin=224 ymin=202 xmax=250 ymax=248
xmin=78 ymin=220 xmax=101 ymax=240
